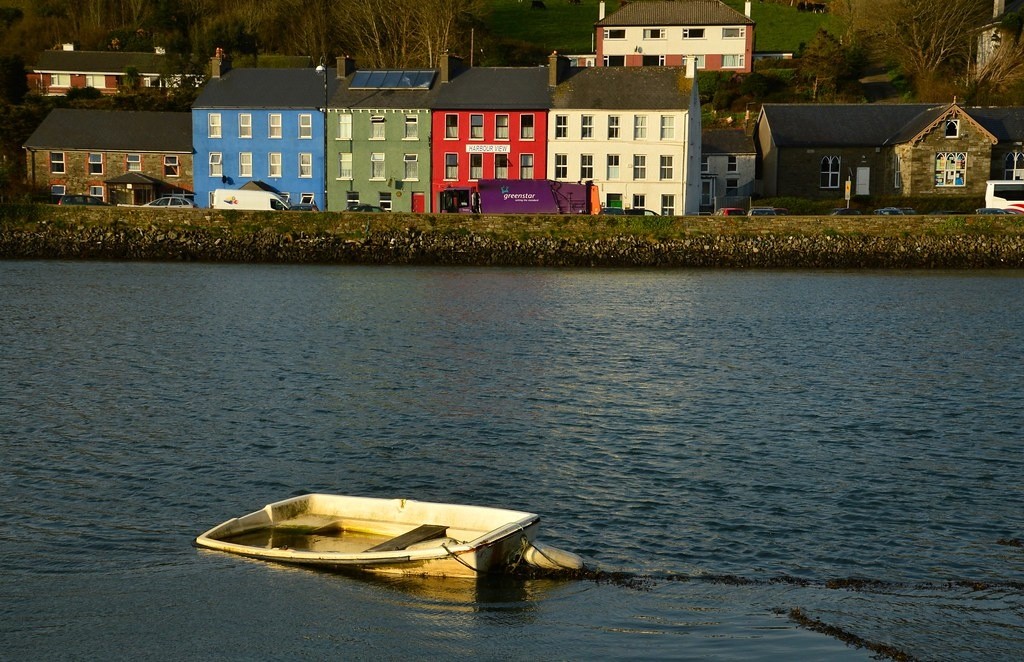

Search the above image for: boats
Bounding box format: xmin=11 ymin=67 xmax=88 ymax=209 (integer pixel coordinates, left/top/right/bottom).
xmin=194 ymin=491 xmax=587 ymax=582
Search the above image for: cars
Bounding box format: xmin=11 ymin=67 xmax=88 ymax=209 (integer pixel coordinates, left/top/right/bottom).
xmin=773 ymin=208 xmax=788 ymax=216
xmin=288 ymin=204 xmax=319 ymax=211
xmin=41 ymin=193 xmax=113 ymax=206
xmin=712 ymin=207 xmax=746 ymax=216
xmin=353 ymin=203 xmax=387 ymax=213
xmin=873 ymin=206 xmax=904 ymax=215
xmin=831 ymin=208 xmax=863 ymax=215
xmin=141 ymin=196 xmax=200 ymax=209
xmin=598 ymin=207 xmax=625 ymax=216
xmin=974 ymin=207 xmax=1017 ymax=215
xmin=748 ymin=209 xmax=776 ymax=215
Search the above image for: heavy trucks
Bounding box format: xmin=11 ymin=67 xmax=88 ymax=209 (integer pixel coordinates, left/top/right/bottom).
xmin=441 ymin=178 xmax=602 ymax=216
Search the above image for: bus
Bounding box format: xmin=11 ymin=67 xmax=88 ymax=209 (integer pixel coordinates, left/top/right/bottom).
xmin=985 ymin=180 xmax=1024 ymax=215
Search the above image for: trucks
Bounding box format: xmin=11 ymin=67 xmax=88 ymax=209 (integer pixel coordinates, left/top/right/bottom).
xmin=212 ymin=189 xmax=290 ymax=211
